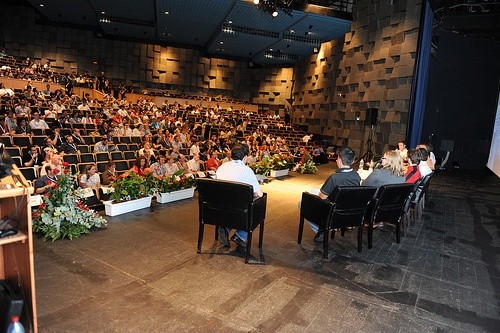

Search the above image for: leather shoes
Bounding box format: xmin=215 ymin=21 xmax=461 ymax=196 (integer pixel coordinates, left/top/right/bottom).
xmin=231 ymin=235 xmax=246 ymax=248
xmin=218 ymin=228 xmax=231 ymax=248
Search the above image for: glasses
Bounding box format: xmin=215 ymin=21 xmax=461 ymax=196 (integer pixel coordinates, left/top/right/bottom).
xmin=381 ymin=157 xmax=386 ymax=160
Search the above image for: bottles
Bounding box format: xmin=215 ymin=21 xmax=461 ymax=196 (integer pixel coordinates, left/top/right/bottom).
xmin=360 ymin=159 xmax=364 ymax=171
xmin=7 ymin=315 xmax=25 ymax=333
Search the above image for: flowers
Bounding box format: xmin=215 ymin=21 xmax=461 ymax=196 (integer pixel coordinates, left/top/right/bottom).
xmin=107 ymin=166 xmax=158 ymax=202
xmin=249 ymin=158 xmax=271 ymax=177
xmin=268 ymin=152 xmax=297 ymax=170
xmin=154 ymin=168 xmax=195 ymax=197
xmin=291 ymin=150 xmax=318 ymax=174
xmin=32 ymin=162 xmax=108 ymax=242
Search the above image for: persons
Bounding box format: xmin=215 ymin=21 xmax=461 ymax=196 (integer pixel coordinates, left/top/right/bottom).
xmin=214 ymin=143 xmax=264 ymax=247
xmin=0 ymin=54 xmax=337 ymax=211
xmin=299 ymin=146 xmax=362 ymax=241
xmin=359 ymin=140 xmax=437 ymax=229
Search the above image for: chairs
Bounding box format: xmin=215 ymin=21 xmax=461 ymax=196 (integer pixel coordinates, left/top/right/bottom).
xmin=341 ymin=183 xmax=415 ymax=249
xmin=298 ymin=185 xmax=377 ymax=259
xmin=379 ymin=171 xmax=435 ymax=237
xmin=0 ymin=56 xmax=338 ymax=225
xmin=196 ymin=178 xmax=267 ymax=263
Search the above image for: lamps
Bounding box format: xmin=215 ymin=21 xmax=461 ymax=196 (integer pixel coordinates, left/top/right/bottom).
xmin=252 ymin=0 xmax=293 ymax=18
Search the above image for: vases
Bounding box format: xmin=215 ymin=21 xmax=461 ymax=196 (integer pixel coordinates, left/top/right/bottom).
xmin=157 ymin=186 xmax=196 ymax=204
xmin=270 ymin=169 xmax=289 ymax=178
xmin=103 ymin=193 xmax=153 ymax=216
xmin=256 ymin=174 xmax=267 ymax=182
xmin=327 ymin=157 xmax=338 ymax=169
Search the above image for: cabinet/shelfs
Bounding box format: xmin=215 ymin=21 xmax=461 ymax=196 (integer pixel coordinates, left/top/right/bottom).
xmin=0 ymin=187 xmax=38 ymax=333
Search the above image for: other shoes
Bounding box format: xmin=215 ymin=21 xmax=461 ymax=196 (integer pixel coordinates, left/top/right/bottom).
xmin=313 ymin=232 xmax=324 ymax=241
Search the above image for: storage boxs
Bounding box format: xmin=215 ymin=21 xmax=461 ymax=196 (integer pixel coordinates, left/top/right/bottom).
xmin=0 ymin=280 xmax=24 ymax=333
